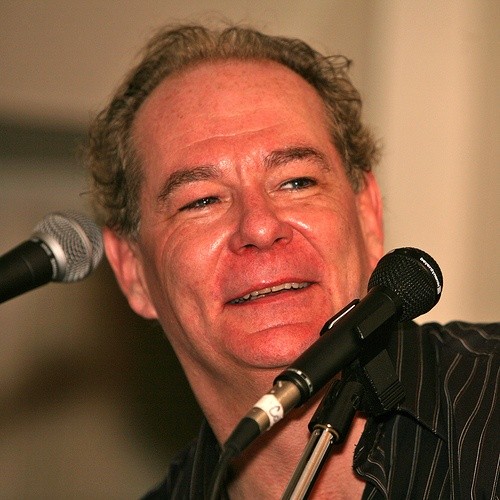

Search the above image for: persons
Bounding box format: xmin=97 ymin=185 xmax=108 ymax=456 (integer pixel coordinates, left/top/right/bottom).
xmin=78 ymin=9 xmax=500 ymax=500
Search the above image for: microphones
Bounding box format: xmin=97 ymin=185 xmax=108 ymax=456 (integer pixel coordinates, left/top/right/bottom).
xmin=222 ymin=244 xmax=443 ymax=453
xmin=0 ymin=207 xmax=104 ymax=306
